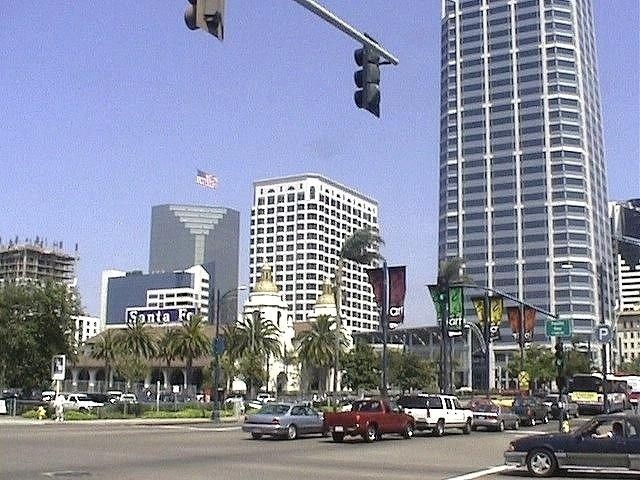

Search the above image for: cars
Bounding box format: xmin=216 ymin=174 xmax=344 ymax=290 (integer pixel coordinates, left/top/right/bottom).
xmin=401 ymin=393 xmax=580 ymax=437
xmin=41 ymin=391 xmax=138 ymax=415
xmin=503 ymin=414 xmax=640 ymax=477
xmin=242 ymin=402 xmax=323 ymax=440
xmin=224 ymin=393 xmax=276 ymax=409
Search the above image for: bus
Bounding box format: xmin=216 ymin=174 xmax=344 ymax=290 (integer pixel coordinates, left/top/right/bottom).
xmin=567 ymin=373 xmax=628 ymax=415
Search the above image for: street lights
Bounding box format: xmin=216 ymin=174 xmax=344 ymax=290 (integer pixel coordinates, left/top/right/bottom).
xmin=433 ymin=293 xmax=448 ymax=393
xmin=486 ymin=290 xmax=493 ymax=395
xmin=561 ymin=264 xmax=607 ymax=374
xmin=366 ymin=248 xmax=389 ymax=388
xmin=214 ymin=286 xmax=247 ymax=423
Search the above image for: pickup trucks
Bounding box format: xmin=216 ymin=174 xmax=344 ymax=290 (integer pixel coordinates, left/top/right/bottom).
xmin=323 ymin=398 xmax=416 ymax=443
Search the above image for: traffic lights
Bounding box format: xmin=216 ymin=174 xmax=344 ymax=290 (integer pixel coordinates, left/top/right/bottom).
xmin=184 ymin=0 xmax=226 ymax=41
xmin=354 ymin=44 xmax=381 ymax=118
xmin=555 ymin=343 xmax=564 ymax=367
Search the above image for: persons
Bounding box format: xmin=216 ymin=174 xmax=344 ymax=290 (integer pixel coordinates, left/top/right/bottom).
xmin=590 ymin=421 xmax=626 ymax=439
xmin=559 ymin=406 xmax=571 ymax=433
xmin=55 ymin=394 xmax=65 ymax=423
